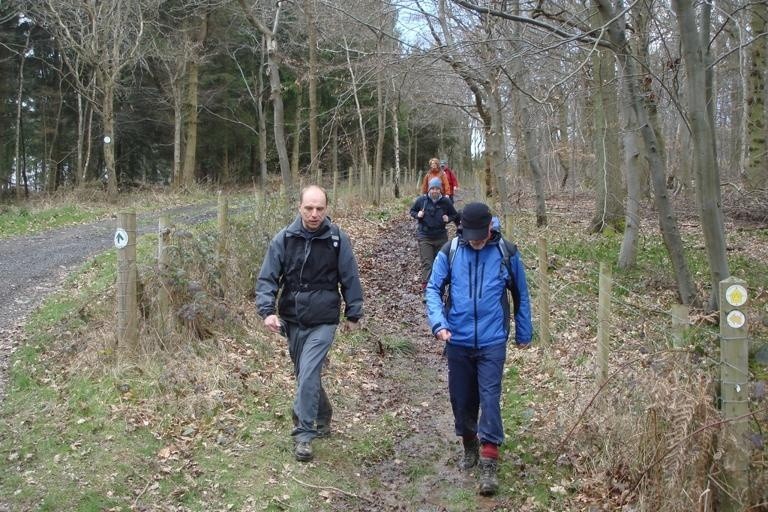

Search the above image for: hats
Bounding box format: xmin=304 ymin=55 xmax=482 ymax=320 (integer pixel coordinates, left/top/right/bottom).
xmin=461 ymin=203 xmax=491 ymax=241
xmin=429 ymin=177 xmax=442 ymax=190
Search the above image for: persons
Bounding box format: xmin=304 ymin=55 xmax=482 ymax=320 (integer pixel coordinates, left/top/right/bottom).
xmin=409 ymin=177 xmax=457 ymax=289
xmin=424 ymin=203 xmax=533 ymax=495
xmin=422 ymin=158 xmax=450 ymax=198
xmin=254 ymin=184 xmax=364 ymax=462
xmin=440 ymin=159 xmax=459 ymax=204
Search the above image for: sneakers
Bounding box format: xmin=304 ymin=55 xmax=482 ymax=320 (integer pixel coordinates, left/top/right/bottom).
xmin=316 ymin=424 xmax=330 ymax=435
xmin=296 ymin=439 xmax=313 ymax=461
xmin=461 ymin=440 xmax=478 ymax=469
xmin=478 ymin=459 xmax=498 ymax=495
